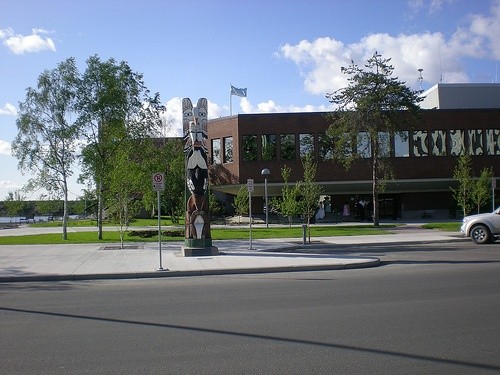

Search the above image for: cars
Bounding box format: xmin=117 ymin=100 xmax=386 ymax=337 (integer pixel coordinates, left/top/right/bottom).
xmin=460 ymin=206 xmax=499 ymax=245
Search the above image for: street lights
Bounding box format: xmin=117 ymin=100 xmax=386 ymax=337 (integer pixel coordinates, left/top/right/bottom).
xmin=261 ymin=168 xmax=270 ymax=229
